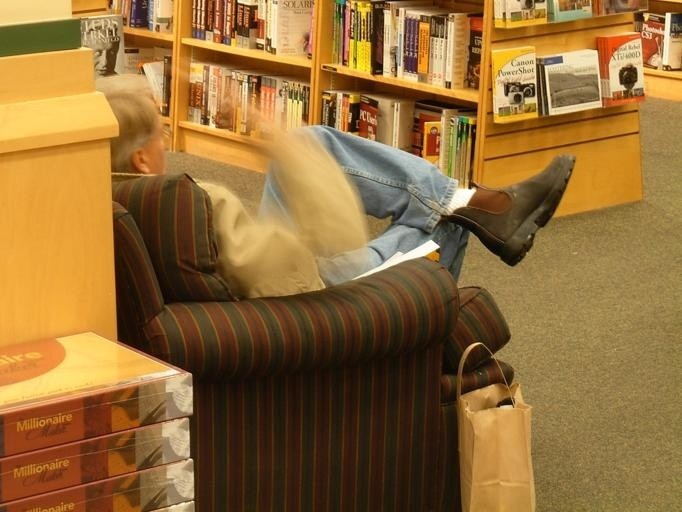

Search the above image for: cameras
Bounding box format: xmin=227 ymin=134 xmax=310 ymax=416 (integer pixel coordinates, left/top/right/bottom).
xmin=619 ymin=66 xmax=638 ymax=85
xmin=504 ymin=80 xmax=536 ymax=105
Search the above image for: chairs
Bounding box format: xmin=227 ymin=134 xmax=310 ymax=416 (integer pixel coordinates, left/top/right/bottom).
xmin=113 ymin=199 xmax=514 ymax=512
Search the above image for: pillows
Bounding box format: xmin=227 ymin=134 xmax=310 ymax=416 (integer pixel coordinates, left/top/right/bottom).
xmin=111 ymin=169 xmax=240 ymax=304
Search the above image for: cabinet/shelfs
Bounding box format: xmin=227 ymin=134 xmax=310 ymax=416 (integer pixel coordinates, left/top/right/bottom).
xmin=113 ymin=2 xmax=681 ymax=217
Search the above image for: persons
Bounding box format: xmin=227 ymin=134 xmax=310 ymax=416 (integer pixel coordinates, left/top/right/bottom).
xmin=95 ymin=73 xmax=577 ymax=301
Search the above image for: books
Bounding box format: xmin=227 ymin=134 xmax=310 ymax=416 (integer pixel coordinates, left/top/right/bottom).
xmin=0 ymin=330 xmax=195 ymax=511
xmin=491 ymin=0 xmax=682 ymax=125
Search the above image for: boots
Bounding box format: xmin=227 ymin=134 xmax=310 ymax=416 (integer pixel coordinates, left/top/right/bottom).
xmin=444 ymin=154 xmax=577 ymax=268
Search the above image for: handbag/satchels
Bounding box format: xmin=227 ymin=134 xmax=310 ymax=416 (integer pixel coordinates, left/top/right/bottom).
xmin=457 ymin=381 xmax=536 ymax=512
